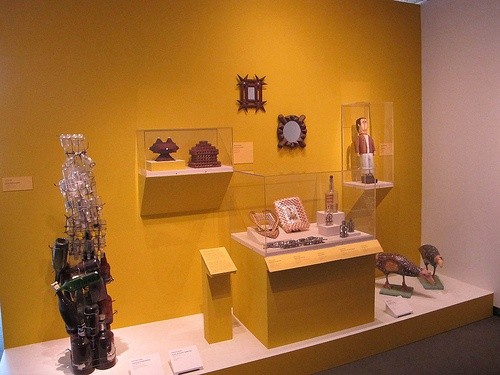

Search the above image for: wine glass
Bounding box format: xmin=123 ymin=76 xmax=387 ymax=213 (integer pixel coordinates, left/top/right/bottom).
xmin=57 ymin=134 xmax=105 ymax=259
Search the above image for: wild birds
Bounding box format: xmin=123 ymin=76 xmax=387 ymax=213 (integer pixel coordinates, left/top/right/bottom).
xmin=418 ymin=244 xmax=444 ymax=280
xmin=375 ymin=253 xmax=437 ymax=294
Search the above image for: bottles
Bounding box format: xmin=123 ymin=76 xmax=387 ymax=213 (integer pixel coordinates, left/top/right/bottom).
xmin=347 ymin=218 xmax=353 ymax=232
xmin=52 ymin=230 xmax=117 ymax=375
xmin=340 ymin=219 xmax=348 ymax=238
xmin=324 ymin=176 xmax=339 ymax=212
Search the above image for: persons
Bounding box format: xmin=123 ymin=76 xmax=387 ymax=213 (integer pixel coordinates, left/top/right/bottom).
xmin=354 ymin=117 xmax=377 ymax=184
